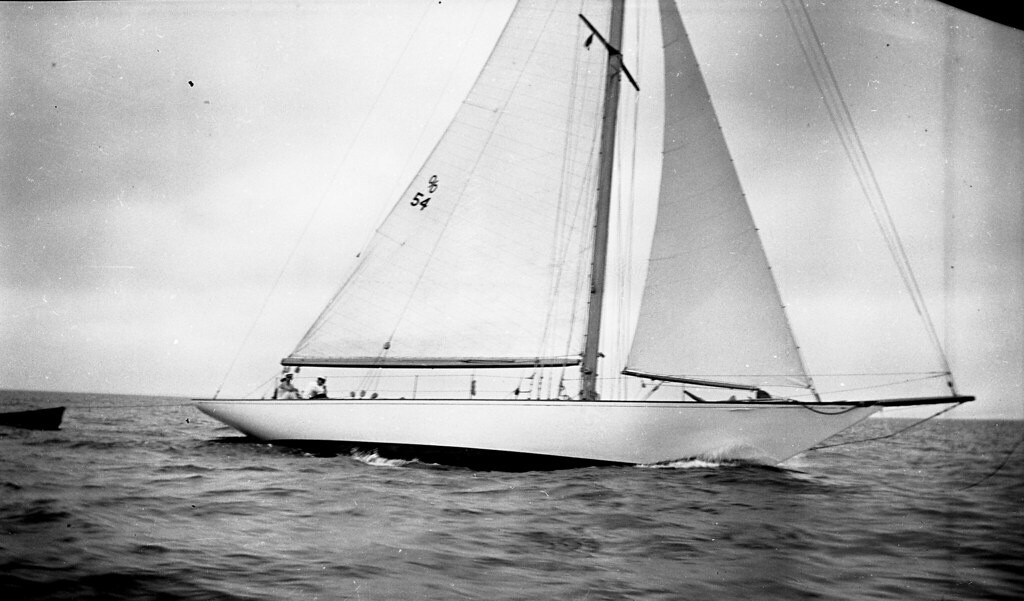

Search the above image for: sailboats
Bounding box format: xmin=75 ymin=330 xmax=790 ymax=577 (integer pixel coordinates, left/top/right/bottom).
xmin=195 ymin=0 xmax=980 ymax=472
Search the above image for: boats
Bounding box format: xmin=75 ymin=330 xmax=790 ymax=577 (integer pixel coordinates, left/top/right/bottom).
xmin=0 ymin=404 xmax=69 ymax=430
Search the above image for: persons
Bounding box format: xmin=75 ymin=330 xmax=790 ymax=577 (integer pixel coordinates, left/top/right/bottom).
xmin=309 ymin=377 xmax=327 ymax=396
xmin=273 ymin=373 xmax=304 ymax=400
